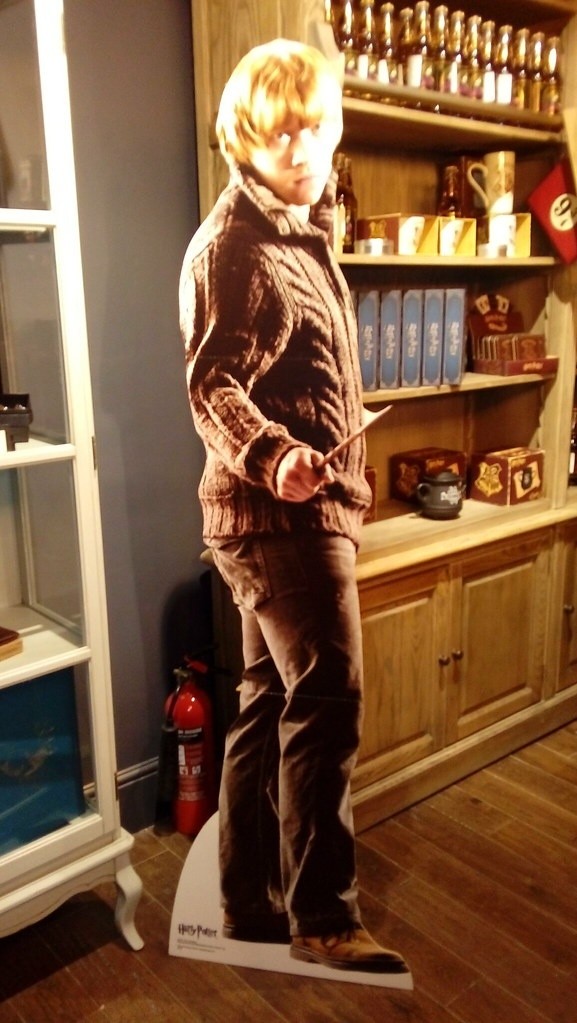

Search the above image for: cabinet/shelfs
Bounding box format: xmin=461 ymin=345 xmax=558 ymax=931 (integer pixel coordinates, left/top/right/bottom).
xmin=188 ymin=0 xmax=577 ymax=837
xmin=0 ymin=0 xmax=148 ymax=960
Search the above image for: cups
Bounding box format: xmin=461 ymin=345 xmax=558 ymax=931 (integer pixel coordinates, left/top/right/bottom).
xmin=416 ymin=467 xmax=468 ymax=519
xmin=468 ymin=150 xmax=516 ymax=216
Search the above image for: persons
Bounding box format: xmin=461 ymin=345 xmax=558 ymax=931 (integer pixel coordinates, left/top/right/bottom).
xmin=175 ymin=35 xmax=412 ymax=974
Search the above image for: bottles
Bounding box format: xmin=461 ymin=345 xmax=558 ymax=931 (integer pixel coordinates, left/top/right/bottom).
xmin=328 ymin=151 xmax=358 ymax=254
xmin=330 ymin=0 xmax=562 ymax=126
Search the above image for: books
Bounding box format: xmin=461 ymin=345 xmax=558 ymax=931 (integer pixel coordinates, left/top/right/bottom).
xmin=350 ymin=288 xmax=468 ymax=391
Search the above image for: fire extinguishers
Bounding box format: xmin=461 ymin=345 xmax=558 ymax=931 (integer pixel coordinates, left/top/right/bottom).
xmin=154 ymin=660 xmax=217 ymax=837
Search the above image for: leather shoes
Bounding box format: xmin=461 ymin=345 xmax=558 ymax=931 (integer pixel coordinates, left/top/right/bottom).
xmin=286 ymin=916 xmax=411 ymax=974
xmin=219 ymin=903 xmax=293 ymax=945
